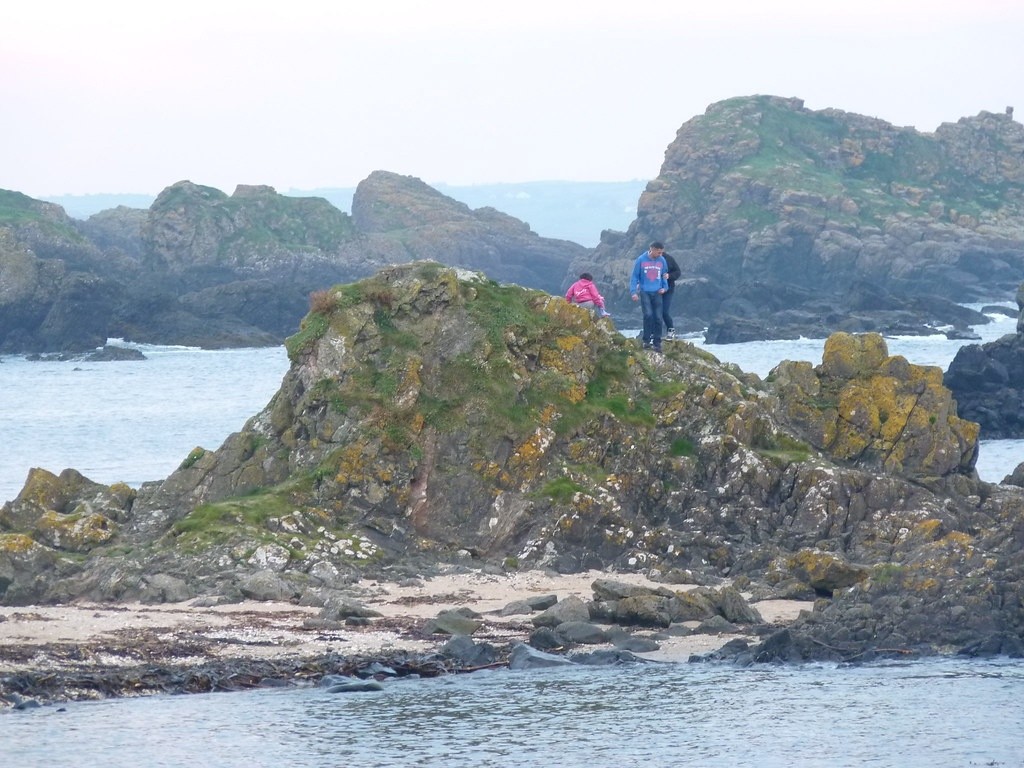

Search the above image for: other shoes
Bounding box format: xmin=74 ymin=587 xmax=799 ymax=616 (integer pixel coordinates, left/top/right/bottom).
xmin=665 ymin=328 xmax=676 ymax=341
xmin=652 ymin=345 xmax=662 ymax=354
xmin=649 ymin=334 xmax=654 ymax=344
xmin=643 ymin=343 xmax=651 ymax=350
xmin=598 ymin=307 xmax=609 ymax=316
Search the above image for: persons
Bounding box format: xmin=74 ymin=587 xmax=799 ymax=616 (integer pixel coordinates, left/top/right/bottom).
xmin=566 ymin=273 xmax=611 ymax=316
xmin=629 ymin=241 xmax=681 ymax=353
